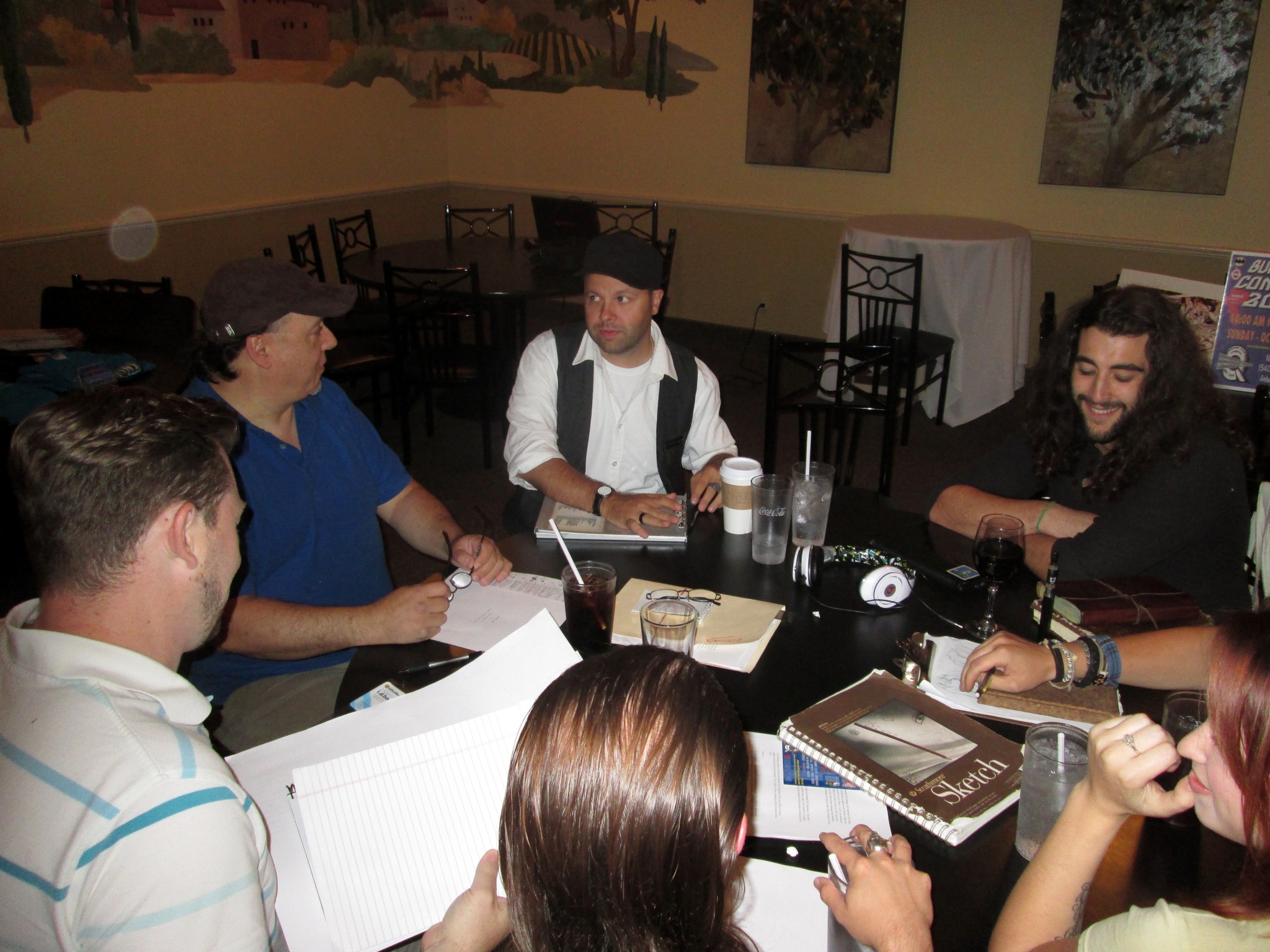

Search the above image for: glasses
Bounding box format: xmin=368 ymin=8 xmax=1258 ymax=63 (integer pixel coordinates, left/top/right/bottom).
xmin=443 ymin=507 xmax=487 ymax=601
xmin=647 ymin=587 xmax=722 ymax=606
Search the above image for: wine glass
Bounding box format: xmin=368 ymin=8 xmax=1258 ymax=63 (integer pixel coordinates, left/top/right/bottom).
xmin=965 ymin=513 xmax=1026 ymax=640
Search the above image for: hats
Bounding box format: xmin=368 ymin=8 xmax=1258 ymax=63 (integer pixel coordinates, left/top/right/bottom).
xmin=206 ymin=256 xmax=357 ymax=343
xmin=583 ymin=231 xmax=667 ymax=292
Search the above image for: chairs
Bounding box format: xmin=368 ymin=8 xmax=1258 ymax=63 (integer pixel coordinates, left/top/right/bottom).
xmin=40 ymin=196 xmax=677 ymax=471
xmin=764 ymin=243 xmax=956 ymax=496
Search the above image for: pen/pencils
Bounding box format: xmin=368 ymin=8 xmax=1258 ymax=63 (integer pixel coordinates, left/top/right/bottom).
xmin=975 ymin=667 xmax=996 ymax=698
xmin=399 ymin=650 xmax=485 ymax=674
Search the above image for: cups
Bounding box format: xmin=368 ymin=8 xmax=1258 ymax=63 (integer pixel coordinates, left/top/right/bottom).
xmin=639 ymin=599 xmax=699 ymax=657
xmin=751 ymin=474 xmax=796 ymax=565
xmin=1160 ymin=691 xmax=1208 ymax=827
xmin=561 ymin=560 xmax=617 ymax=653
xmin=719 ymin=456 xmax=764 ymax=534
xmin=828 ymin=837 xmax=915 ymax=952
xmin=792 ymin=460 xmax=836 ymax=546
xmin=1015 ymin=722 xmax=1091 ymax=861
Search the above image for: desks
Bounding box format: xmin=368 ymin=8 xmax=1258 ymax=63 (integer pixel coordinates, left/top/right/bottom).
xmin=340 ymin=238 xmax=586 ymax=417
xmin=336 ymin=510 xmax=1270 ymax=952
xmin=817 ymin=214 xmax=1032 ymax=428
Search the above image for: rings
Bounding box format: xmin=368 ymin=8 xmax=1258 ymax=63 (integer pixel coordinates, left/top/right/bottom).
xmin=708 ymin=482 xmax=722 ymax=493
xmin=639 ymin=511 xmax=648 ymax=525
xmin=1122 ymin=732 xmax=1142 ymax=757
xmin=865 ymin=830 xmax=890 ymax=856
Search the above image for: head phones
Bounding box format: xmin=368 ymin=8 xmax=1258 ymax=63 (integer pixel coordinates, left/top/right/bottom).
xmin=789 ymin=543 xmax=916 ymax=608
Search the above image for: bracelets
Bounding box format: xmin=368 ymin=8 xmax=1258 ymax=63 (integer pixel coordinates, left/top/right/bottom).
xmin=1038 ymin=633 xmax=1122 ymax=692
xmin=1036 ymin=500 xmax=1057 ymax=535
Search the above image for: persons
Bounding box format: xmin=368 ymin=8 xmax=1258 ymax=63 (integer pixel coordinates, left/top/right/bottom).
xmin=178 ymin=256 xmax=514 ymax=753
xmin=1 ymin=386 xmax=516 ymax=952
xmin=504 ymin=227 xmax=738 ymax=540
xmin=497 ymin=645 xmax=933 ymax=951
xmin=922 ymin=623 xmax=1269 ymax=951
xmin=924 ymin=283 xmax=1253 ymax=613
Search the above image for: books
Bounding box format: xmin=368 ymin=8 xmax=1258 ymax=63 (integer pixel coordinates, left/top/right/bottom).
xmin=775 ymin=666 xmax=1026 ymax=848
xmin=610 ymin=578 xmax=787 ymax=674
xmin=978 ymin=679 xmax=1121 ymax=724
xmin=1030 ymin=576 xmax=1200 ymax=642
xmin=534 ymin=493 xmax=689 ymax=543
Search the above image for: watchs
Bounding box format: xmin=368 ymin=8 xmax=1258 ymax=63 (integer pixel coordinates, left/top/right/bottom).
xmin=592 ymin=485 xmax=613 ymax=517
